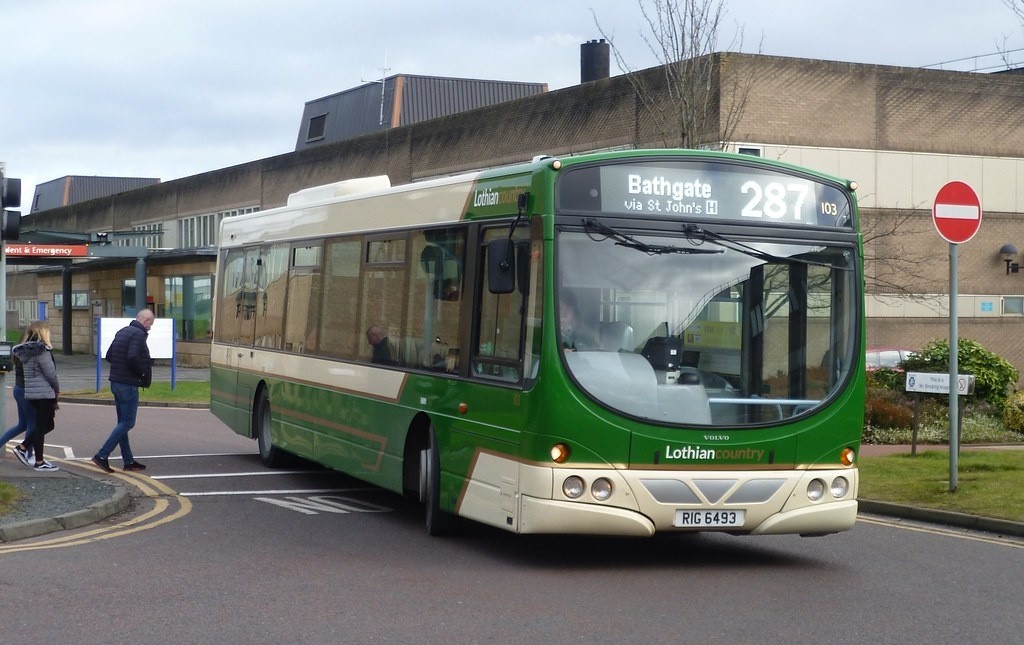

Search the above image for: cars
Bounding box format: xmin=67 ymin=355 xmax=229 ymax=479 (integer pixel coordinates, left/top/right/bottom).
xmin=865 ymin=347 xmax=930 ymax=372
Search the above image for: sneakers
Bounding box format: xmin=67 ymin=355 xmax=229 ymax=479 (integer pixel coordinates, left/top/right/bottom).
xmin=91 ymin=455 xmax=115 ymax=473
xmin=34 ymin=461 xmax=59 ymax=472
xmin=123 ymin=461 xmax=146 ymax=471
xmin=13 ymin=445 xmax=29 ymax=467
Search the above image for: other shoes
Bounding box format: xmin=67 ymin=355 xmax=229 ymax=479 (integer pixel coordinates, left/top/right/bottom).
xmin=28 ymin=456 xmax=35 ymax=463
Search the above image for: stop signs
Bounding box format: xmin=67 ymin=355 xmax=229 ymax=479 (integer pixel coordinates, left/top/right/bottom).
xmin=933 ymin=181 xmax=984 ymax=244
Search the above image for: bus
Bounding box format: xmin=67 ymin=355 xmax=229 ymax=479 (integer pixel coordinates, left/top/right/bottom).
xmin=209 ymin=148 xmax=868 ymax=543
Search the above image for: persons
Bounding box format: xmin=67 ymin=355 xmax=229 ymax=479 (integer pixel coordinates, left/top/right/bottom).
xmin=361 ymin=325 xmax=403 ymax=367
xmin=0 ymin=320 xmax=60 ymax=472
xmin=556 ymin=290 xmax=616 ymax=354
xmin=91 ymin=309 xmax=154 ymax=473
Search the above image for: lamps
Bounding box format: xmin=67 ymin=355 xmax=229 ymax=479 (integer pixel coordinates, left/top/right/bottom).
xmin=1000 ymin=243 xmax=1024 ymax=275
